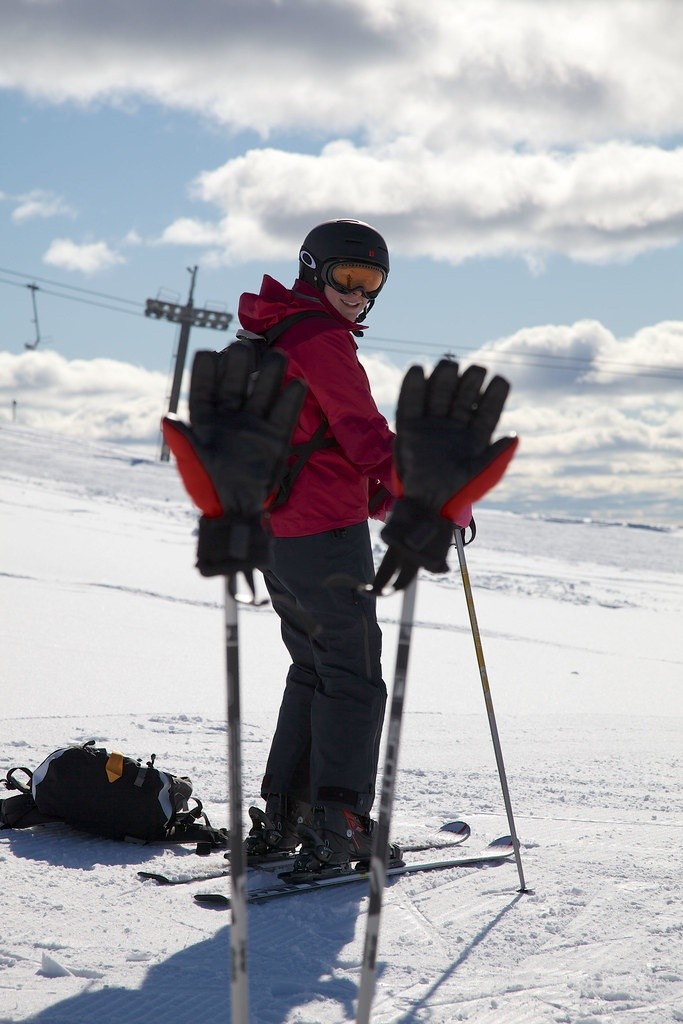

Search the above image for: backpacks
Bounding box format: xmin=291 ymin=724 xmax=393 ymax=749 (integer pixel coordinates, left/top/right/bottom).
xmin=0 ymin=740 xmax=228 ymax=856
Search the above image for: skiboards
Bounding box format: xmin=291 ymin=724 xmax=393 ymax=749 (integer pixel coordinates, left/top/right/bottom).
xmin=135 ymin=816 xmax=521 ymax=907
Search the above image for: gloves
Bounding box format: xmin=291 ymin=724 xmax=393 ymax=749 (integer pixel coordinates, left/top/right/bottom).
xmin=161 ymin=342 xmax=306 ymax=576
xmin=380 ymin=358 xmax=520 ymax=572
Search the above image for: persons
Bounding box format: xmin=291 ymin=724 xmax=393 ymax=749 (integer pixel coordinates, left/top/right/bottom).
xmin=220 ymin=218 xmax=404 ymax=876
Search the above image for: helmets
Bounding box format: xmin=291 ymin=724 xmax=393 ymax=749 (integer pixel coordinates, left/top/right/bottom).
xmin=299 ymin=218 xmax=390 ymax=300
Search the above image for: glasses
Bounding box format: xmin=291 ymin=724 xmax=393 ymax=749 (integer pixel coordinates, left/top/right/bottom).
xmin=320 ymin=261 xmax=387 ymax=300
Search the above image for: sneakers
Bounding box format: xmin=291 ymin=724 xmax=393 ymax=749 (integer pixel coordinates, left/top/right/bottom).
xmin=308 ymin=803 xmax=403 ymax=863
xmin=264 ymin=803 xmax=308 ymax=846
